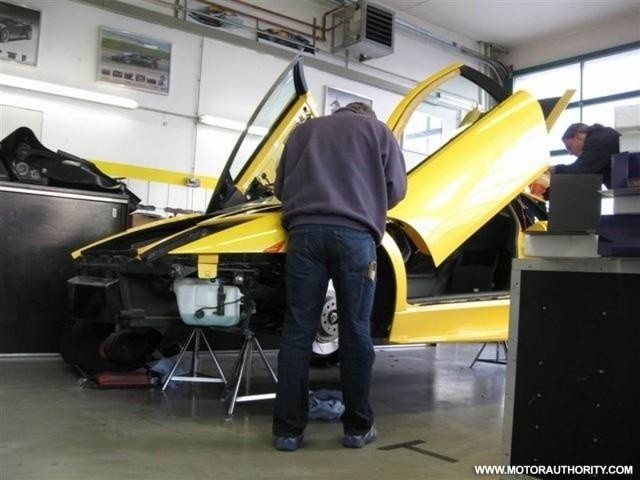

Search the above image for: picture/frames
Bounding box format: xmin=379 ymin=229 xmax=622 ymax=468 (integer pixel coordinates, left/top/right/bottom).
xmin=0 ymin=2 xmax=174 ymax=95
xmin=324 ymin=86 xmax=374 ymax=117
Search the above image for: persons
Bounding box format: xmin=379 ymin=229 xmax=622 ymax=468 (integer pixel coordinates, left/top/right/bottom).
xmin=271 ymin=99 xmax=408 ymax=451
xmin=545 ymin=122 xmax=623 ymax=190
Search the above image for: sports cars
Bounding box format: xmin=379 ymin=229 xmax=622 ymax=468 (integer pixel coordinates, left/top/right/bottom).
xmin=55 ymin=43 xmax=561 ymax=382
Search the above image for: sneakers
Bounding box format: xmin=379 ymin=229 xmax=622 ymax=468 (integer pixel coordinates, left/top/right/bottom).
xmin=276 ymin=436 xmax=303 ymax=451
xmin=344 ymin=426 xmax=376 ymax=447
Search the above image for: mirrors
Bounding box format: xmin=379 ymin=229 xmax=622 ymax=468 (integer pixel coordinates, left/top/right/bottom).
xmin=400 ymin=110 xmax=444 ymax=156
